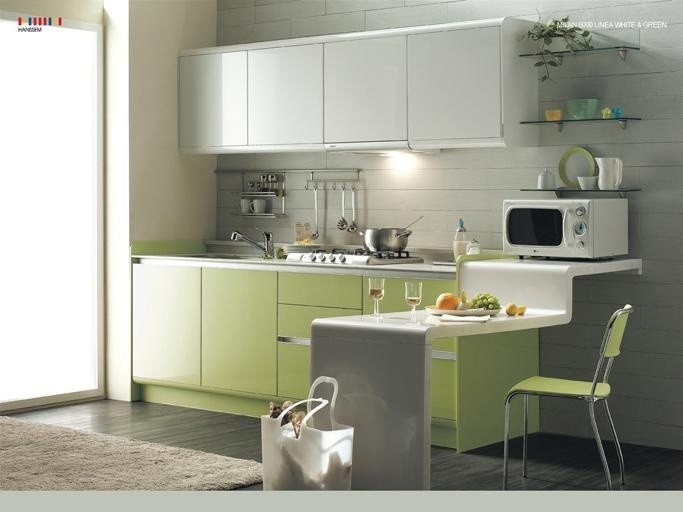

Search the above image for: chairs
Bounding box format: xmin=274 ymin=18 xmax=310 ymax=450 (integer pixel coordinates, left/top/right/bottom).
xmin=502 ymin=303 xmax=635 ymax=491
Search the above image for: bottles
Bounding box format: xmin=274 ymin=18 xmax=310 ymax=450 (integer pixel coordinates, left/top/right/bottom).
xmin=537 ymin=168 xmax=555 ymax=190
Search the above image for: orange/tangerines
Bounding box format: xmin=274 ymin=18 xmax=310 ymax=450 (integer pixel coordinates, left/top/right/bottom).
xmin=506 ymin=303 xmax=518 ymax=316
xmin=517 ymin=305 xmax=526 ymax=315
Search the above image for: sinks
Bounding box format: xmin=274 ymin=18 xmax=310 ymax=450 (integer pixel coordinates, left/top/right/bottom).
xmin=179 ymin=253 xmax=245 ymax=259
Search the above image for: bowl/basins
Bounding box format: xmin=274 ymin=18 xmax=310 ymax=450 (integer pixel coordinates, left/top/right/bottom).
xmin=577 ymin=176 xmax=598 ymax=190
xmin=566 ymin=99 xmax=598 ymax=120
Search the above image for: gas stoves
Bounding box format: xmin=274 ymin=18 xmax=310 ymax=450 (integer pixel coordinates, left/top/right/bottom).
xmin=287 ymin=247 xmax=425 ymax=265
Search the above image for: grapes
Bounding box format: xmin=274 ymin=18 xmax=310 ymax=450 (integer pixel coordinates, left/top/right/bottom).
xmin=472 ymin=291 xmax=501 ymax=310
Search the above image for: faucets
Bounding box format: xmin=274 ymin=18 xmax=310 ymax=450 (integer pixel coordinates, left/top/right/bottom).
xmin=230 ymin=227 xmax=274 ymax=259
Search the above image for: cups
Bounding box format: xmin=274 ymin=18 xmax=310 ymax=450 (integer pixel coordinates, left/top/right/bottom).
xmin=240 ymin=199 xmax=250 ymax=212
xmin=544 ymin=110 xmax=561 ymax=120
xmin=250 ymin=199 xmax=266 ymax=213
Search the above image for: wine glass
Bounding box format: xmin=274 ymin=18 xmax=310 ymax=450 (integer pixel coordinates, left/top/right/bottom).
xmin=404 ymin=281 xmax=423 ymax=326
xmin=369 ymin=277 xmax=385 ymax=319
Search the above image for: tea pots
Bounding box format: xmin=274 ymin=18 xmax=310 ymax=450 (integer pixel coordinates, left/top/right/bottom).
xmin=594 ymin=157 xmax=624 ymax=191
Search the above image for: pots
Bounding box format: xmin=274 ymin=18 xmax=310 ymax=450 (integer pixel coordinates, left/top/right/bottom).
xmin=358 ymin=227 xmax=413 ymax=253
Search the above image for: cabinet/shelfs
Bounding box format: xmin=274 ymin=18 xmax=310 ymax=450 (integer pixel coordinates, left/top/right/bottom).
xmin=322 ymin=15 xmax=540 ymax=150
xmin=362 ymin=271 xmax=541 ymax=454
xmin=175 ymin=34 xmax=326 ymax=155
xmin=276 ymin=262 xmax=364 ymax=434
xmin=131 ymin=257 xmax=277 ymax=423
xmin=515 ymin=27 xmax=642 ymax=199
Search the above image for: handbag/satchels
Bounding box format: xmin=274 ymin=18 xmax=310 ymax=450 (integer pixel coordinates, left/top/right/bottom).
xmin=260 ymin=377 xmax=354 ymax=489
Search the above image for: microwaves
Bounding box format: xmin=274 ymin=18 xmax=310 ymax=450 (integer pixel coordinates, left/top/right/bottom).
xmin=501 ymin=198 xmax=630 ymax=260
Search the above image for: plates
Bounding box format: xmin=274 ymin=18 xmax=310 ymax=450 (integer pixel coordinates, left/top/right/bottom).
xmin=558 ymin=146 xmax=595 ymax=189
xmin=425 ymin=306 xmax=501 ymax=316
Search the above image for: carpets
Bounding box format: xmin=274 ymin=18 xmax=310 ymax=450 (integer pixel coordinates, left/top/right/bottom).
xmin=0 ymin=411 xmax=264 ymax=492
